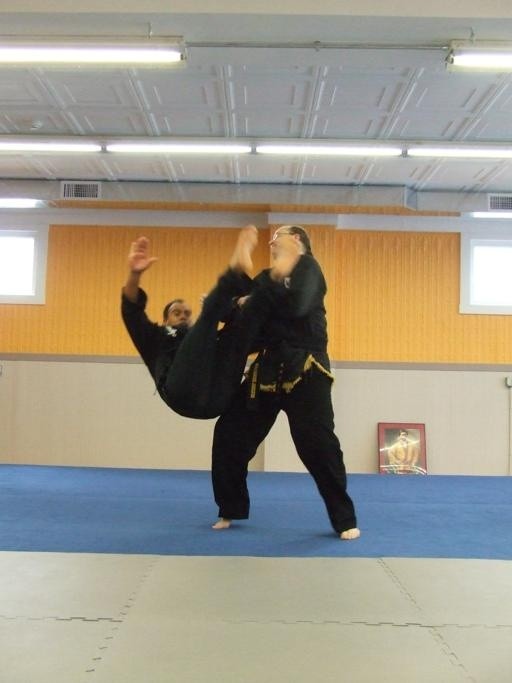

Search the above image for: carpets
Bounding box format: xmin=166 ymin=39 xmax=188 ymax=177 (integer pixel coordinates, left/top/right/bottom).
xmin=0 ymin=465 xmax=512 ymax=556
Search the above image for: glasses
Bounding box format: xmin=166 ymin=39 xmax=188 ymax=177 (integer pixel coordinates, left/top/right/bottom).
xmin=274 ymin=232 xmax=294 ymax=240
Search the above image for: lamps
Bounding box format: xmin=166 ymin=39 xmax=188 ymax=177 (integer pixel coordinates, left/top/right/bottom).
xmin=250 ymin=143 xmax=404 ymax=157
xmin=2 ymin=138 xmax=104 ymax=155
xmin=444 ymin=37 xmax=512 ymax=69
xmin=0 ymin=35 xmax=188 ymax=64
xmin=404 ymin=145 xmax=511 ymax=159
xmin=101 ymin=140 xmax=253 ymax=157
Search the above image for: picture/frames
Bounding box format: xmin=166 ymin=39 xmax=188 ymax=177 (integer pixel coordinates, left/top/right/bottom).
xmin=378 ymin=422 xmax=427 ymax=475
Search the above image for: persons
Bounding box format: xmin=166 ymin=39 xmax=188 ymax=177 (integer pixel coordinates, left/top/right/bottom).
xmin=388 ymin=429 xmax=418 ymax=474
xmin=200 ymin=227 xmax=362 ymax=540
xmin=120 ymin=222 xmax=301 ymax=419
